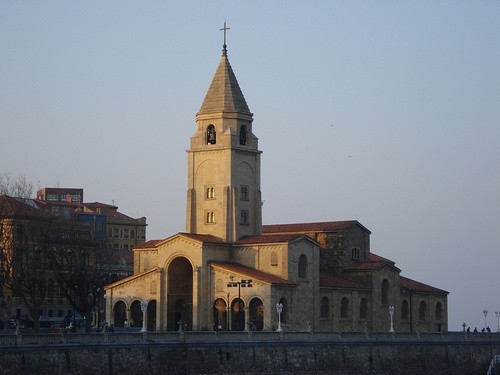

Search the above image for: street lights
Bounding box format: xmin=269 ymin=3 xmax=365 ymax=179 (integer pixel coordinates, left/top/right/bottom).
xmin=388 ymin=306 xmax=395 ymax=332
xmin=482 ymin=310 xmax=489 ymax=332
xmin=275 ymin=303 xmax=284 ymax=332
xmin=495 ymin=311 xmax=500 ymax=332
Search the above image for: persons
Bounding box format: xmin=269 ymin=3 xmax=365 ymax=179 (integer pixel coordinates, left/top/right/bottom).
xmin=466 ymin=327 xmax=470 ymax=332
xmin=48 ymin=319 xmax=74 ymax=337
xmin=98 ymin=320 xmax=113 ymax=334
xmin=8 ymin=314 xmax=33 ymax=335
xmin=130 ymin=318 xmax=135 ymax=326
xmin=481 ymin=328 xmax=485 ymax=332
xmin=176 ymin=319 xmax=192 ymax=330
xmin=214 ymin=320 xmax=225 ymax=332
xmin=474 ymin=327 xmax=477 ymax=332
xmin=250 ymin=317 xmax=263 ymax=331
xmin=231 ymin=316 xmax=244 ymax=330
xmin=486 ymin=326 xmax=491 ymax=333
xmin=124 ymin=319 xmax=128 ymax=326
